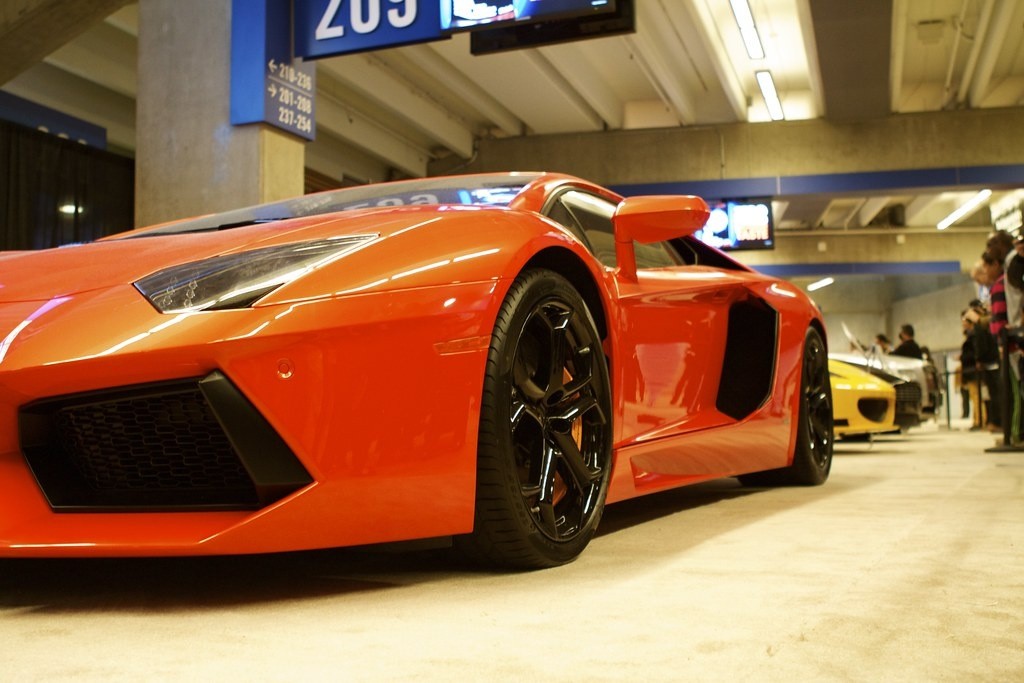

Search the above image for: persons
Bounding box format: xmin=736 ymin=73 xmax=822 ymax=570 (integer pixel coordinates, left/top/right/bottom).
xmin=849 ymin=324 xmax=940 ymax=422
xmin=956 ymin=230 xmax=1024 ymax=450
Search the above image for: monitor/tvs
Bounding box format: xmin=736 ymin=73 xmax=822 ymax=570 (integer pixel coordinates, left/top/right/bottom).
xmin=472 ymin=0 xmax=637 ymax=56
xmin=694 ymin=197 xmax=774 ymax=251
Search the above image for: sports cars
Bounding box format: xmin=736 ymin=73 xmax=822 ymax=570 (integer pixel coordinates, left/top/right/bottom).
xmin=0 ymin=169 xmax=838 ymax=573
xmin=828 ymin=344 xmax=944 ymax=444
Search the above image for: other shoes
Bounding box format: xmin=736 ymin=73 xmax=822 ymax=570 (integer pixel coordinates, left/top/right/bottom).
xmin=969 ymin=425 xmax=981 ymax=430
